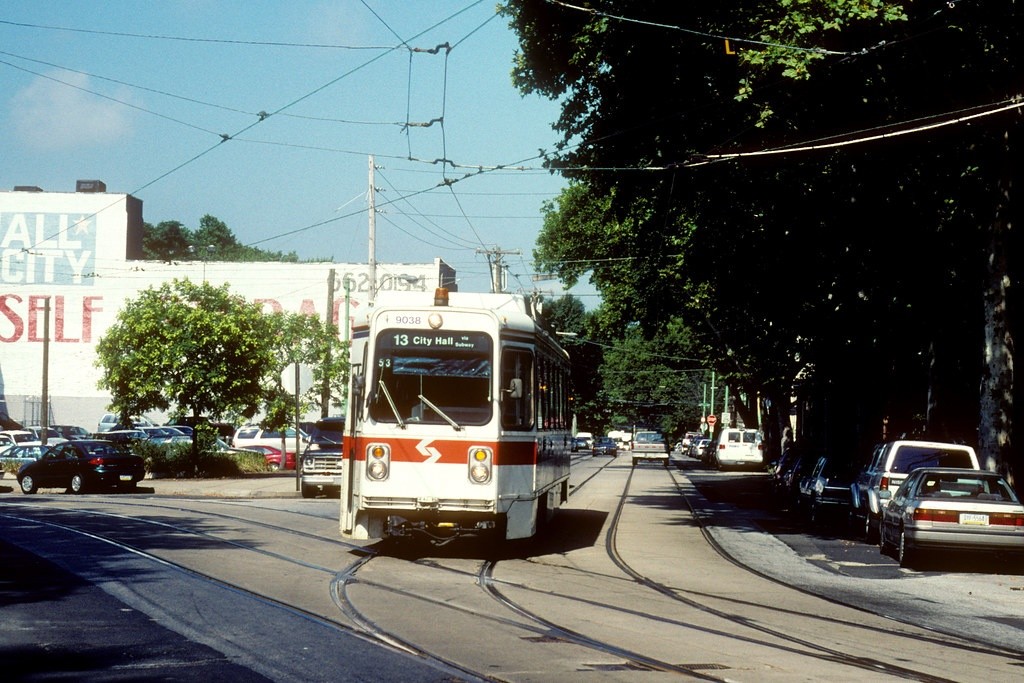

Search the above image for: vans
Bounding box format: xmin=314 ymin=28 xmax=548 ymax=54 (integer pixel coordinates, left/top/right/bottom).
xmin=163 ymin=417 xmax=210 ymax=428
xmin=716 ymin=428 xmax=764 ymax=471
xmin=232 ymin=423 xmax=321 ymax=452
xmin=97 ymin=414 xmax=159 ymax=432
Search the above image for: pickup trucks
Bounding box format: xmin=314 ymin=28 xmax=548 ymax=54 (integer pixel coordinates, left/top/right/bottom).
xmin=632 ymin=431 xmax=670 ymax=467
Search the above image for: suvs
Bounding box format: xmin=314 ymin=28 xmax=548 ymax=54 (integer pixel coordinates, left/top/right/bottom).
xmin=300 ymin=417 xmax=346 ymax=499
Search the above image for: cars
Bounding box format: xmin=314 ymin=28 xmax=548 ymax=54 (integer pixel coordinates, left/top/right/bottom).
xmin=850 ymin=439 xmax=981 ymax=544
xmin=772 ymin=447 xmax=867 ymax=523
xmin=0 ymin=445 xmax=74 ymax=471
xmin=692 ymin=439 xmax=712 ymax=459
xmin=238 ymin=445 xmax=296 ymax=470
xmin=571 ymin=433 xmax=632 ymax=453
xmin=17 ymin=440 xmax=146 ymax=494
xmin=592 ymin=437 xmax=617 ymax=458
xmin=702 ymin=441 xmax=716 ymax=467
xmin=0 ymin=423 xmax=242 ymax=454
xmin=881 ymin=467 xmax=1024 ymax=572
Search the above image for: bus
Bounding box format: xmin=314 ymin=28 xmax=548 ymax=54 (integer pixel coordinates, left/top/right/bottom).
xmin=337 ymin=289 xmax=572 ymax=546
